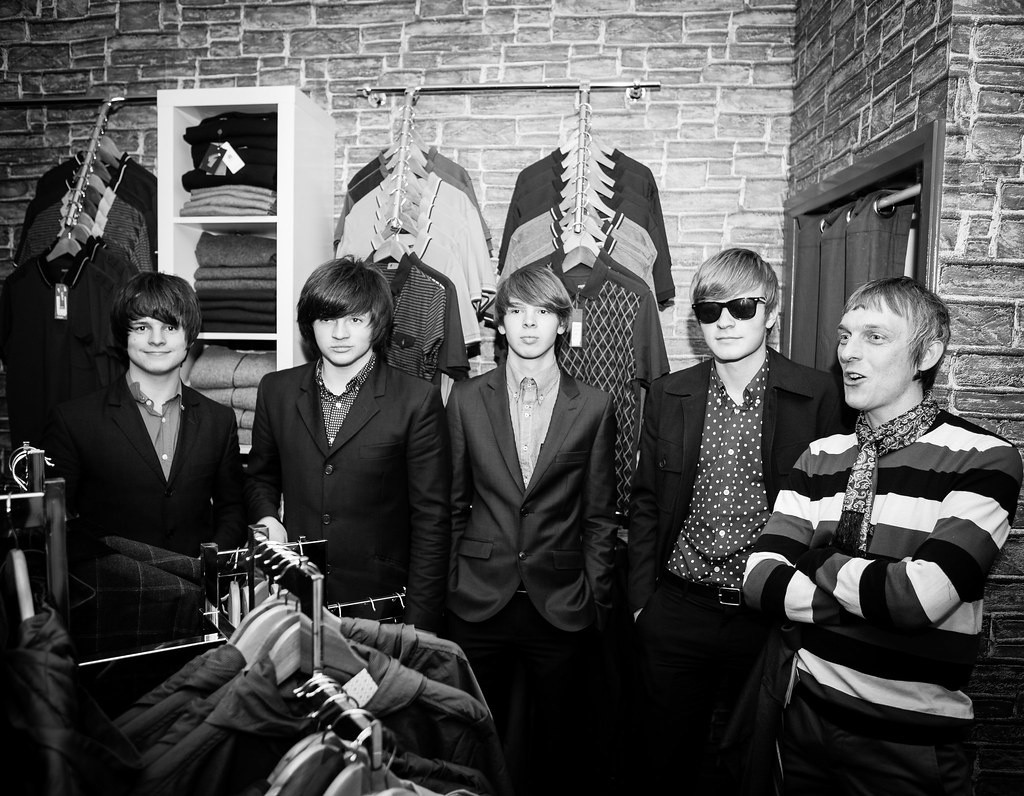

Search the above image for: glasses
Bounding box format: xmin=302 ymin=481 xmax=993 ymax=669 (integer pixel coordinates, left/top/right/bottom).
xmin=692 ymin=293 xmax=769 ymax=324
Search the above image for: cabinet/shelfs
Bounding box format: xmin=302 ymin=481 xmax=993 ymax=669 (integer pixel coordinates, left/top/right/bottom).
xmin=156 ymin=88 xmax=336 ymax=454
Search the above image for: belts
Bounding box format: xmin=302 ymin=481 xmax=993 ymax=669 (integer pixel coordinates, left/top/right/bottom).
xmin=660 ymin=570 xmax=752 ymax=608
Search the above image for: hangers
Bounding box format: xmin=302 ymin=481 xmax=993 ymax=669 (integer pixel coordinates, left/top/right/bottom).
xmin=560 ymin=102 xmax=615 ymax=274
xmin=228 ymin=542 xmax=406 ymax=796
xmin=7 ymin=444 xmax=55 ymax=622
xmin=373 ymin=105 xmax=430 ymax=265
xmin=47 ymin=102 xmax=123 ymax=262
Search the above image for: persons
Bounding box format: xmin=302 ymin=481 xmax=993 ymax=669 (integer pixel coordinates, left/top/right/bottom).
xmin=41 ymin=270 xmax=243 ymax=558
xmin=445 ymin=266 xmax=617 ymax=796
xmin=742 ymin=276 xmax=1024 ymax=796
xmin=246 ymin=255 xmax=451 ymax=631
xmin=624 ymin=248 xmax=841 ymax=796
